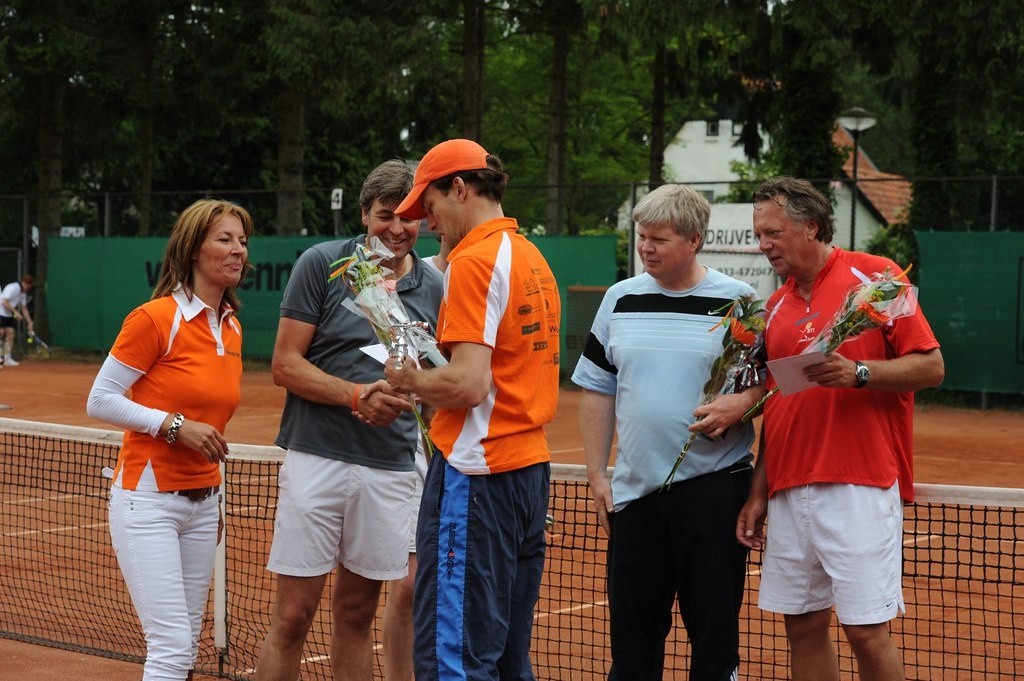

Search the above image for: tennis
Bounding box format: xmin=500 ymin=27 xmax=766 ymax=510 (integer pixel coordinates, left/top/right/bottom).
xmin=27 ymin=338 xmax=33 ymax=344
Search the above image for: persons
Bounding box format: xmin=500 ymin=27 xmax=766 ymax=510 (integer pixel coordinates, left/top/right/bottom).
xmin=376 ymin=138 xmax=564 ymax=680
xmin=0 ymin=275 xmax=34 ymax=364
xmin=248 ymin=159 xmax=449 ymax=681
xmin=383 ymin=230 xmax=446 ymax=679
xmin=570 ymin=176 xmax=769 ymax=680
xmin=87 ymin=200 xmax=253 ymax=680
xmin=735 ymin=168 xmax=943 ymax=681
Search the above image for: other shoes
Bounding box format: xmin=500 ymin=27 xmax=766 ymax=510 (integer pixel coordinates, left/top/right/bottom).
xmin=3 ymin=358 xmax=19 ymax=366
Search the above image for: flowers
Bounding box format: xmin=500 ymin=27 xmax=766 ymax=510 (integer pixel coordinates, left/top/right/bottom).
xmin=741 ymin=262 xmax=921 ymax=423
xmin=658 ymin=294 xmax=774 ymax=492
xmin=328 ymin=233 xmax=441 ymax=459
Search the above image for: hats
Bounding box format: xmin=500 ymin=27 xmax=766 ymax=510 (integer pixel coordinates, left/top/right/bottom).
xmin=394 ymin=139 xmax=500 ymax=221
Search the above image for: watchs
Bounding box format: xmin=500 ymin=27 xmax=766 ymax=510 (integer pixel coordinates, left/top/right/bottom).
xmin=855 ymin=359 xmax=873 ymax=389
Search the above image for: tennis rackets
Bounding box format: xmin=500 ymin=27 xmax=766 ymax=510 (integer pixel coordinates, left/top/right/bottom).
xmin=13 ymin=314 xmax=52 ymax=361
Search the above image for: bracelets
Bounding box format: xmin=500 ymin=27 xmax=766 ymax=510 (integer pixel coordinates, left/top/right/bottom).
xmin=164 ymin=410 xmax=186 ymax=444
xmin=351 ymin=384 xmax=361 ymax=409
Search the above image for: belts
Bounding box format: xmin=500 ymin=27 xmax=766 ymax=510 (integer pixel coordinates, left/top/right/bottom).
xmin=165 ymin=484 xmax=219 ymax=502
